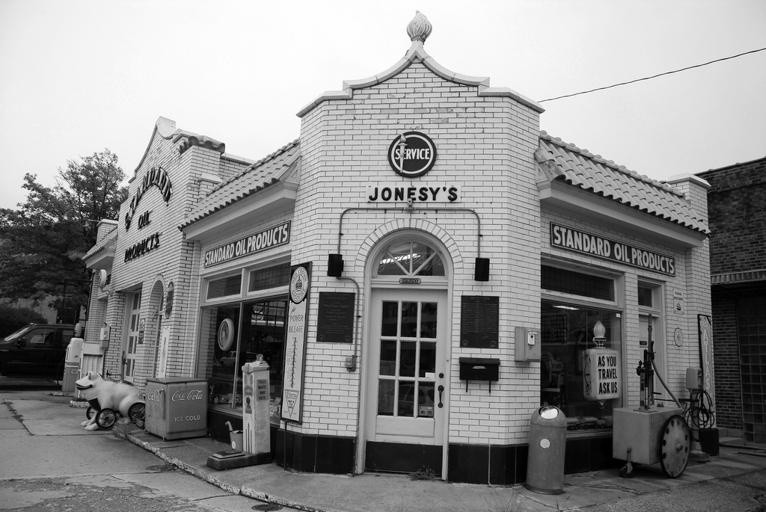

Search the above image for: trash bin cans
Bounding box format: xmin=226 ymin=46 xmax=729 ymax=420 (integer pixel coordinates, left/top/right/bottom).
xmin=523 ymin=405 xmax=567 ymax=495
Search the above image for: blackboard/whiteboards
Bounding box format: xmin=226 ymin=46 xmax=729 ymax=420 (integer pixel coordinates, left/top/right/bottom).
xmin=460 ymin=295 xmax=499 ymax=348
xmin=317 ymin=292 xmax=355 ymax=343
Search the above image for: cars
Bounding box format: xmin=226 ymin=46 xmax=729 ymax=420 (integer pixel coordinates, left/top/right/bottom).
xmin=1 ymin=323 xmax=85 ymax=381
